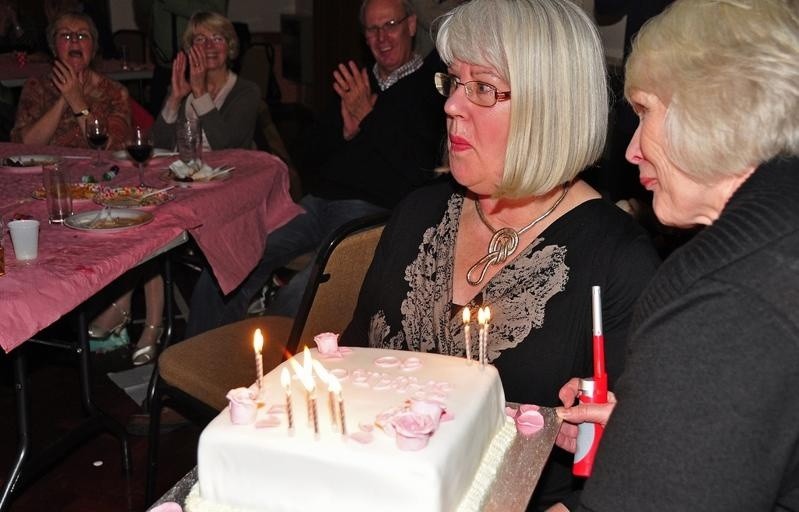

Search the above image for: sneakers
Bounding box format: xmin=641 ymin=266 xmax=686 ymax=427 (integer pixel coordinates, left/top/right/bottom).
xmin=128 ymin=404 xmax=187 ymax=438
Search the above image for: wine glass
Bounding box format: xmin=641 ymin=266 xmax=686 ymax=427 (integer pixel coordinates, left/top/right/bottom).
xmin=85 ymin=118 xmax=114 ymax=168
xmin=123 ymin=127 xmax=154 ymax=189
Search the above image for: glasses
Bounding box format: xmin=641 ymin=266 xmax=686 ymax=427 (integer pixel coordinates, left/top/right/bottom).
xmin=362 ymin=14 xmax=409 ymax=39
xmin=433 ymin=69 xmax=512 ymax=108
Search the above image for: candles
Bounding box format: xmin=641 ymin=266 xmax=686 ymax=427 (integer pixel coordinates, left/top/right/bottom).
xmin=251 ymin=299 xmax=494 ymax=435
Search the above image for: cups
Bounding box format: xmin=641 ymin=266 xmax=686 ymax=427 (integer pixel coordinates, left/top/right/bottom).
xmin=175 ymin=118 xmax=204 ymax=164
xmin=7 ymin=220 xmax=40 ymax=260
xmin=44 ymin=161 xmax=72 ymax=226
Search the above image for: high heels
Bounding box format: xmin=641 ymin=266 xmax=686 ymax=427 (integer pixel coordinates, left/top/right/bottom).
xmin=131 ymin=322 xmax=166 ymax=366
xmin=87 ymin=302 xmax=132 ymax=342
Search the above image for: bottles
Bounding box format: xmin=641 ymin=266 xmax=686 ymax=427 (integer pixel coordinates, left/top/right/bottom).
xmin=104 ymin=165 xmax=120 ymax=181
xmin=80 ymin=176 xmax=98 ymax=184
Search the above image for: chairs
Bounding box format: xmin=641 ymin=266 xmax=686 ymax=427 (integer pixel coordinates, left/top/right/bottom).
xmin=143 ymin=206 xmax=393 ymax=511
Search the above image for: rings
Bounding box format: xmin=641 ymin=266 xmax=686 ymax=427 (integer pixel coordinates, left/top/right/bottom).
xmin=345 ymin=86 xmax=351 ymax=93
xmin=194 ymin=63 xmax=200 ymax=67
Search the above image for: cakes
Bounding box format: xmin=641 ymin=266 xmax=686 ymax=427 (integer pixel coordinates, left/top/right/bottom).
xmin=185 ymin=332 xmax=517 ymax=512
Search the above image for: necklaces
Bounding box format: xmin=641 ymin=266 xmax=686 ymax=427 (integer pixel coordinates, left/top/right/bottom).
xmin=463 ymin=179 xmax=574 ymax=285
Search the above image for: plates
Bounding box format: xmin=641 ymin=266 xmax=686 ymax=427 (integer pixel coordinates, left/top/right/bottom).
xmin=92 ymin=185 xmax=175 ymax=209
xmin=113 ymin=147 xmax=180 ymax=158
xmin=64 ymin=209 xmax=152 ymax=231
xmin=2 ymin=155 xmax=63 ymax=174
xmin=32 ymin=184 xmax=98 ymax=202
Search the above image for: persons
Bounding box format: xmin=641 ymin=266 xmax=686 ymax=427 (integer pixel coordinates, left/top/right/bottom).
xmin=127 ymin=0 xmax=451 ymax=439
xmin=331 ymin=0 xmax=662 ymax=512
xmin=555 ymin=0 xmax=798 ymax=511
xmin=86 ymin=8 xmax=265 ymax=369
xmin=0 ymin=0 xmax=146 ymax=151
